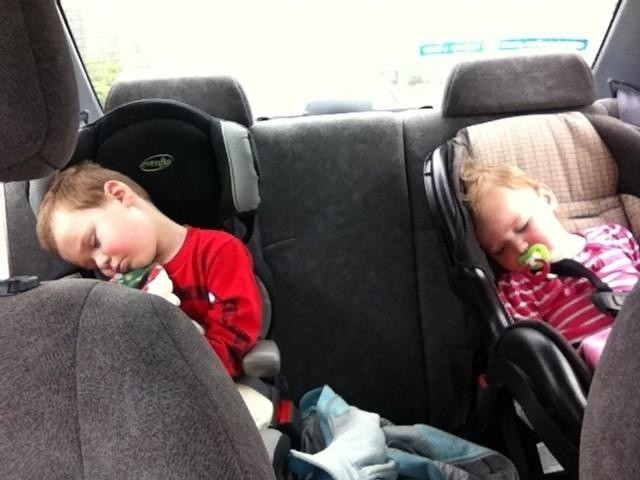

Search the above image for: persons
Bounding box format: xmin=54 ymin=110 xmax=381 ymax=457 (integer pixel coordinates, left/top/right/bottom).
xmin=457 ymin=143 xmax=639 ymax=375
xmin=34 ymin=158 xmax=263 ymax=388
